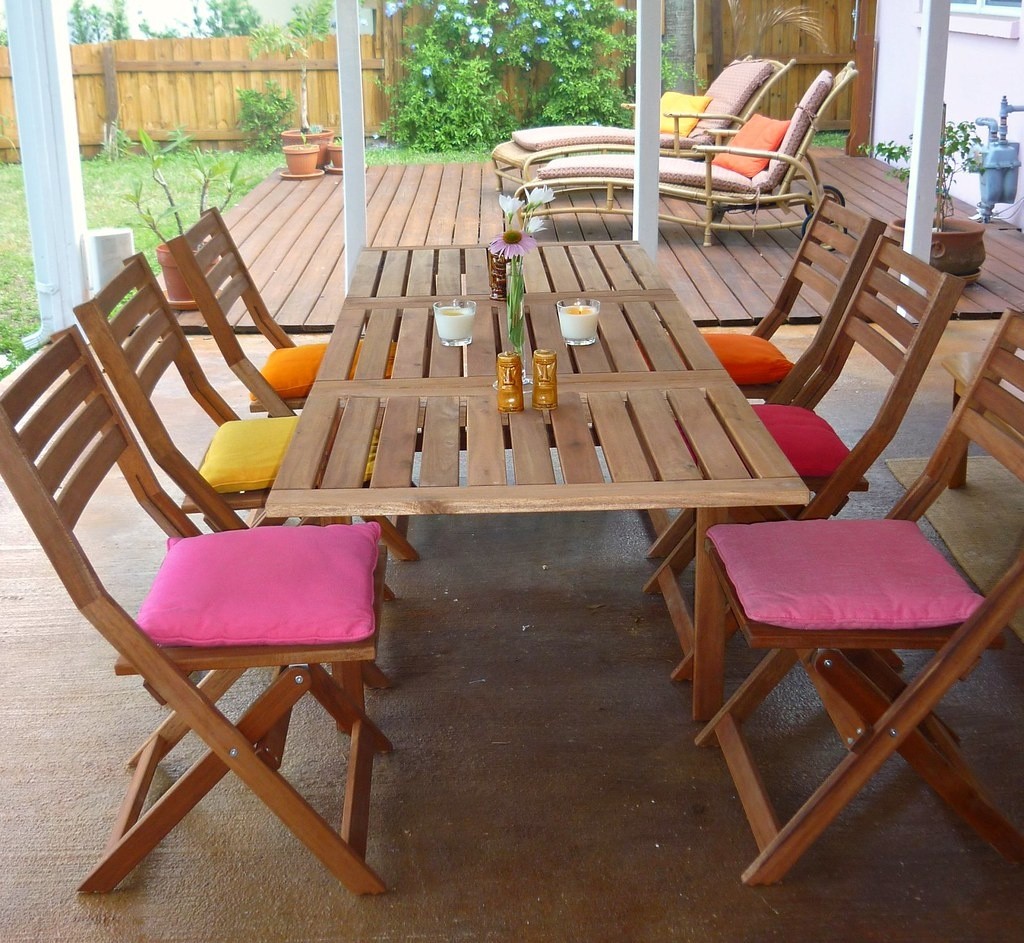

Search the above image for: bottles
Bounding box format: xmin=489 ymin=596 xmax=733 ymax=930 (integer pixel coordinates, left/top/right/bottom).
xmin=533 ymin=349 xmax=558 ymax=410
xmin=496 ymin=352 xmax=524 ymax=413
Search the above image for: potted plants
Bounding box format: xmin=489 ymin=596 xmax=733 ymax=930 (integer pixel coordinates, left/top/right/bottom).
xmin=246 ymin=1 xmax=335 ymax=169
xmin=327 ymin=135 xmax=343 ymax=167
xmin=857 ymin=120 xmax=987 ymax=275
xmin=118 ymin=121 xmax=241 ymax=301
xmin=282 ymin=132 xmax=320 ymax=174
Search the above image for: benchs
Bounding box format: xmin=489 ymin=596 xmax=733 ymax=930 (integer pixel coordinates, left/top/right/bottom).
xmin=491 ymin=54 xmax=798 ymax=192
xmin=512 ymin=54 xmax=857 ymax=245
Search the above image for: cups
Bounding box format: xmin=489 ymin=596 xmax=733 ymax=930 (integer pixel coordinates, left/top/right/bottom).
xmin=433 ymin=300 xmax=477 ymax=347
xmin=556 ymin=297 xmax=600 ymax=347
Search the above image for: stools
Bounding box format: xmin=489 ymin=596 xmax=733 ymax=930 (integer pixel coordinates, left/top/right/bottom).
xmin=941 ymin=348 xmax=1024 ymax=488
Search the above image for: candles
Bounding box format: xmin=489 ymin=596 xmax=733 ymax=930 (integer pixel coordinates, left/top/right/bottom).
xmin=434 ymin=305 xmax=475 ymax=340
xmin=558 ymin=304 xmax=599 ymax=339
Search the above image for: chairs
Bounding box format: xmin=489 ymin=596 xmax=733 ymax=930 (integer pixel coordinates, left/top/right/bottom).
xmin=72 ymin=252 xmax=393 ymax=702
xmin=642 ymin=193 xmax=889 ymax=559
xmin=0 ymin=326 xmax=393 ymax=903
xmin=695 ymin=307 xmax=1024 ymax=883
xmin=643 ymin=234 xmax=967 ymax=685
xmin=164 ymin=204 xmax=419 ymax=563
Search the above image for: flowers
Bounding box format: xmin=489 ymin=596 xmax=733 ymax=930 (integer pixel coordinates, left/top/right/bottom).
xmin=487 ymin=228 xmax=540 ymax=352
xmin=485 ymin=183 xmax=557 ymax=265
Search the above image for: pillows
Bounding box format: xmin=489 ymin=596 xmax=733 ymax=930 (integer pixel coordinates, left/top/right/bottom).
xmin=711 ymin=113 xmax=789 ymax=177
xmin=661 ymin=91 xmax=715 ymax=136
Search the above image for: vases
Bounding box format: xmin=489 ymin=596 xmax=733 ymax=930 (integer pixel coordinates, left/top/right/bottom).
xmin=486 ymin=247 xmax=520 ymax=301
xmin=266 ymin=240 xmax=816 ymax=720
xmin=493 ymin=264 xmax=532 ymax=392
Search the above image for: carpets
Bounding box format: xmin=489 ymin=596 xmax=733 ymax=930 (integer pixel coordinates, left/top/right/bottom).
xmin=885 ymin=453 xmax=1024 ymax=642
